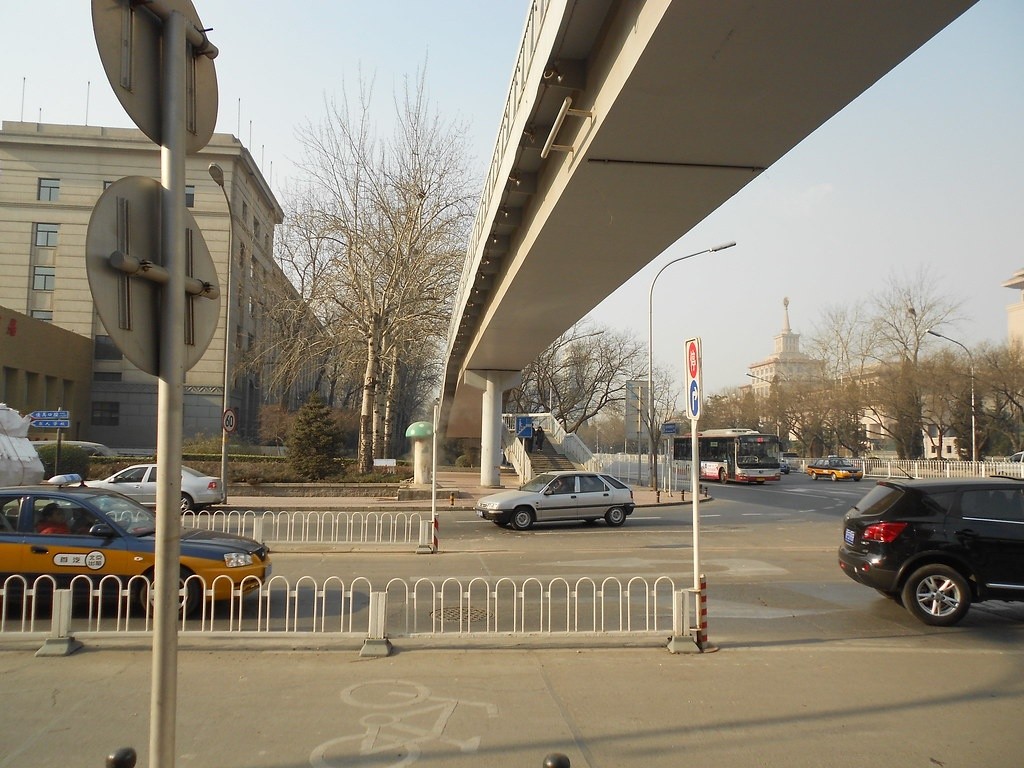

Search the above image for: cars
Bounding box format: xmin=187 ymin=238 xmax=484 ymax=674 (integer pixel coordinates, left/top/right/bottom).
xmin=471 ymin=470 xmax=637 ymax=531
xmin=779 ymin=459 xmax=790 ymax=474
xmin=806 ymin=455 xmax=863 ymax=482
xmin=56 ymin=462 xmax=227 ymax=514
xmin=1006 ymin=451 xmax=1024 ymax=464
xmin=0 ymin=474 xmax=273 ymax=618
xmin=838 ymin=475 xmax=1024 ymax=627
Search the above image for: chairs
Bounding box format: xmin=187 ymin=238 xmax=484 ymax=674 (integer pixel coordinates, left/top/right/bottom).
xmin=33 ymin=511 xmax=42 ymax=533
xmin=63 ymin=508 xmax=75 ymax=526
xmin=990 ymin=491 xmax=1010 ymax=512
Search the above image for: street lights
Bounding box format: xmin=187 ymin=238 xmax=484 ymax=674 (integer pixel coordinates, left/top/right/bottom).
xmin=926 ymin=328 xmax=975 ymax=469
xmin=646 ymin=241 xmax=738 ymax=486
xmin=549 ymin=329 xmax=606 ymax=414
xmin=202 ymin=162 xmax=239 ymax=498
xmin=745 ymin=373 xmax=780 ymax=443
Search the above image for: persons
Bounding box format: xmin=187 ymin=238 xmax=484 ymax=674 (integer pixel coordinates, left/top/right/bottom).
xmin=36 ymin=502 xmax=129 ymax=536
xmin=526 ymin=423 xmax=535 ymax=452
xmin=553 ymin=478 xmax=567 ymax=492
xmin=535 ymin=426 xmax=545 ymax=452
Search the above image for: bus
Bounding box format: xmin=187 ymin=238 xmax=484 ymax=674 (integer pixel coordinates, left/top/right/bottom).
xmin=671 ymin=428 xmax=784 ymax=484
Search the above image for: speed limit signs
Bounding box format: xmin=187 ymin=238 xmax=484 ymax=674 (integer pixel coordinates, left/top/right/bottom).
xmin=222 ymin=409 xmax=236 ymax=432
